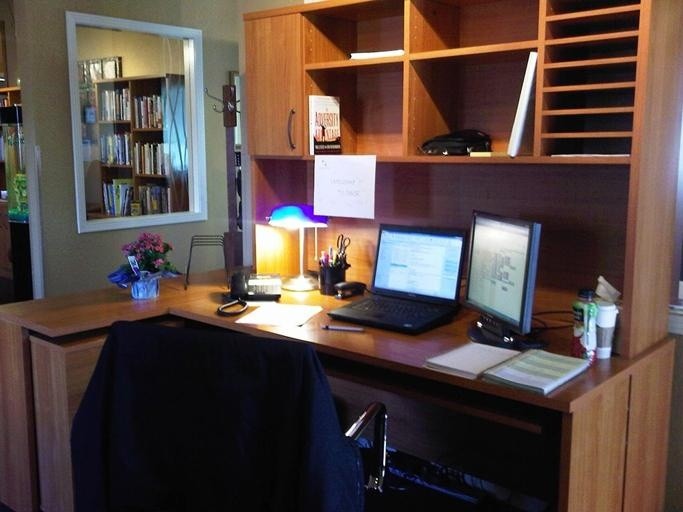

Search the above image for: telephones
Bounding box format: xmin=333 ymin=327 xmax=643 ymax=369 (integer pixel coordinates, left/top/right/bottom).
xmin=230 ymin=272 xmax=281 ymax=301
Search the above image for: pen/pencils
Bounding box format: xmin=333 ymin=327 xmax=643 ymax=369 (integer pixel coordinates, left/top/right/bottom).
xmin=319 ymin=244 xmax=347 ymax=268
xmin=320 ymin=325 xmax=364 ymax=333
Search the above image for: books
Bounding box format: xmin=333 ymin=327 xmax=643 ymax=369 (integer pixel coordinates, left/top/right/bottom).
xmin=486 ymin=347 xmax=590 ymax=396
xmin=347 ymin=49 xmax=403 ymax=59
xmin=308 ymin=96 xmax=341 ymax=155
xmin=504 ymin=51 xmax=537 ymax=159
xmin=422 ymin=341 xmax=523 ymax=381
xmin=96 ymin=82 xmax=169 ymax=216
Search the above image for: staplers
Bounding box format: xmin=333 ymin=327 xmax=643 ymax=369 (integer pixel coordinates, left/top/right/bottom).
xmin=334 ymin=281 xmax=367 ymax=299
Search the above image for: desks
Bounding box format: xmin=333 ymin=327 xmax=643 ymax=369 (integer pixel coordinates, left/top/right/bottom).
xmin=1 ymin=268 xmax=676 ymax=512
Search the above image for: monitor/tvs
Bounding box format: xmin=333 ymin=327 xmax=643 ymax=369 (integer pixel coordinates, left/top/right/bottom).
xmin=464 ymin=209 xmax=550 ymax=350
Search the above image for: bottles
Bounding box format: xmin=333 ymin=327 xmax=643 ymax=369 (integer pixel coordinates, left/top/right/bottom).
xmin=570 ymin=284 xmax=596 ymax=364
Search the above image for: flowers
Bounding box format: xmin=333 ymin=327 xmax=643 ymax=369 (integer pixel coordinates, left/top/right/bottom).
xmin=120 ymin=232 xmax=183 ymax=280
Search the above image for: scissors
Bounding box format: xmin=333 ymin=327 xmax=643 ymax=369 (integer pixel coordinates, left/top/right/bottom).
xmin=337 ymin=234 xmax=351 ymax=254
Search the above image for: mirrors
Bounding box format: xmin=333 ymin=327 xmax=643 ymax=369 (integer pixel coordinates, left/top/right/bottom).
xmin=63 ymin=10 xmax=207 ymax=235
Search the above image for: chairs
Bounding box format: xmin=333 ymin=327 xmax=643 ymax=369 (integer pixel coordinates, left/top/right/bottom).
xmin=104 ymin=320 xmax=389 ymax=512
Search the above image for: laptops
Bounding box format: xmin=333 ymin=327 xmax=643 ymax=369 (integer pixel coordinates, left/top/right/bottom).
xmin=327 ymin=224 xmax=467 ymax=335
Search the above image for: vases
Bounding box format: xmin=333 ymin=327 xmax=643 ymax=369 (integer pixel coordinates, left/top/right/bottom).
xmin=127 ymin=268 xmax=162 ymax=301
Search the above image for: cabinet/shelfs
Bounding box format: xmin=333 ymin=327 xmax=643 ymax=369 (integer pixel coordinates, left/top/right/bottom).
xmin=83 ymin=73 xmax=189 ymax=218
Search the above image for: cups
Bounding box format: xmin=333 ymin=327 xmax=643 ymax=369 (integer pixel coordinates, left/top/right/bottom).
xmin=319 ymin=266 xmax=346 ymax=295
xmin=595 ymin=302 xmax=618 ymax=360
